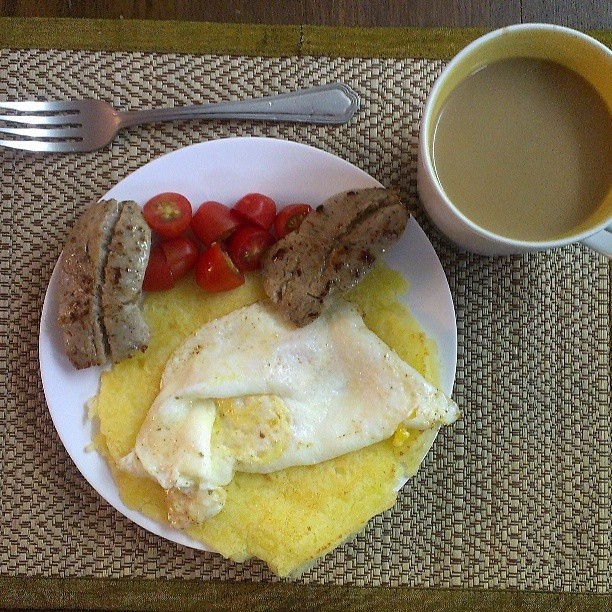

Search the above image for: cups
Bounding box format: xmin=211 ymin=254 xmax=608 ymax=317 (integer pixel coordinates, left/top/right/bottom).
xmin=418 ymin=23 xmax=612 ymax=255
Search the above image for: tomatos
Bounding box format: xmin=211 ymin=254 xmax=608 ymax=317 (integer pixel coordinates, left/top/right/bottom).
xmin=144 ymin=191 xmax=313 ymax=291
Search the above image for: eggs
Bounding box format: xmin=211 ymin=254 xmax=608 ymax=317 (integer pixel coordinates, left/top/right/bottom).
xmin=115 ymin=300 xmax=460 ymax=521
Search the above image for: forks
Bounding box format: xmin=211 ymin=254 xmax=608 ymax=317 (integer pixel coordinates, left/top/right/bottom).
xmin=1 ymin=82 xmax=361 ymax=154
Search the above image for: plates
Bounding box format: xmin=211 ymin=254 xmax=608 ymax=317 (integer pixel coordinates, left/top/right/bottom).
xmin=38 ymin=138 xmax=457 ymax=554
